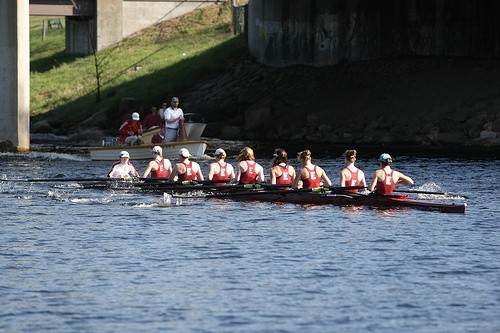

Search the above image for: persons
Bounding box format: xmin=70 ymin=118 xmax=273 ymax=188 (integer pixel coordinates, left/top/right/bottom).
xmin=208 ymin=148 xmax=235 ymax=186
xmin=370 ymin=154 xmax=413 ymax=195
xmin=143 ymin=145 xmax=172 ymax=178
xmin=169 ymin=149 xmax=204 ymax=182
xmin=107 ymin=151 xmax=139 ymax=178
xmin=236 ymin=147 xmax=264 ymax=184
xmin=270 ymin=149 xmax=296 ymax=190
xmin=118 ymin=112 xmax=143 ymax=146
xmin=143 ymin=106 xmax=162 ymax=129
xmin=158 ymin=102 xmax=168 ymax=120
xmin=163 ymin=97 xmax=183 ymax=143
xmin=292 ymin=150 xmax=332 ymax=189
xmin=340 ymin=150 xmax=367 ymax=193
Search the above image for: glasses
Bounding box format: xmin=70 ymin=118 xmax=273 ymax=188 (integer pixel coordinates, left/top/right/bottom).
xmin=152 ymin=108 xmax=158 ymax=110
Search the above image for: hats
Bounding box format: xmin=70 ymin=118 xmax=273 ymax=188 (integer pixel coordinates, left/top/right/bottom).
xmin=118 ymin=151 xmax=129 ymax=158
xmin=153 ymin=145 xmax=162 ymax=152
xmin=172 ymin=97 xmax=178 ymax=102
xmin=132 ymin=112 xmax=140 ymax=120
xmin=163 ymin=103 xmax=167 ymax=106
xmin=214 ymin=148 xmax=226 ymax=158
xmin=179 ymin=148 xmax=190 ymax=157
xmin=378 ymin=153 xmax=393 ymax=163
xmin=273 ymin=152 xmax=278 ymax=157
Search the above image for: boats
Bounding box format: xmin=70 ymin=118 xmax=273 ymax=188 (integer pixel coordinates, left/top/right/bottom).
xmin=50 ymin=178 xmax=468 ymax=217
xmin=88 ymin=112 xmax=210 ymax=160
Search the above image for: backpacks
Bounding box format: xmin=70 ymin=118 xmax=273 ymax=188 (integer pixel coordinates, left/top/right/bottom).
xmin=152 ymin=135 xmax=163 ymax=142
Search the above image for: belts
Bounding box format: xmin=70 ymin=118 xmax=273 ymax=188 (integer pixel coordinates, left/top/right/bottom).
xmin=166 ymin=128 xmax=178 ymax=130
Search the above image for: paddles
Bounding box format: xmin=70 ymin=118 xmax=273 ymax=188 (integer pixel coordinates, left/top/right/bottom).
xmin=113 ymin=184 xmax=293 ymax=194
xmin=155 ymin=186 xmax=364 ymax=198
xmin=0 ymin=177 xmax=168 ymax=182
xmin=369 ymin=187 xmax=468 ymax=199
xmin=50 ymin=179 xmax=231 ymax=189
